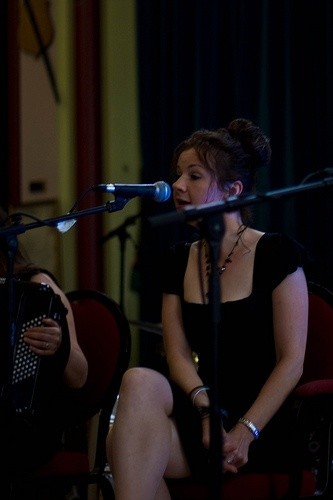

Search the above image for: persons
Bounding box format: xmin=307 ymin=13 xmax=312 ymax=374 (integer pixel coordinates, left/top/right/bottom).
xmin=103 ymin=115 xmax=309 ymax=500
xmin=0 ymin=207 xmax=89 ymax=500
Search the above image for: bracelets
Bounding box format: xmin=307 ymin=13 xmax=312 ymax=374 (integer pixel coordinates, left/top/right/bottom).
xmin=237 ymin=416 xmax=261 ymax=440
xmin=188 ymin=385 xmax=211 ymax=419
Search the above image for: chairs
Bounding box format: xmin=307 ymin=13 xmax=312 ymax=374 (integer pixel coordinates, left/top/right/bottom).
xmin=214 ymin=281 xmax=332 ymax=500
xmin=31 ymin=287 xmax=132 ymax=499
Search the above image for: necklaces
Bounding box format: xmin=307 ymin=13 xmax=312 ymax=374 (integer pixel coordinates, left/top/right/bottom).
xmin=204 ymin=225 xmax=249 ymax=301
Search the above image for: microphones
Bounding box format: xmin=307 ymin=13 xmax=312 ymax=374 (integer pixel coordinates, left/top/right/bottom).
xmin=92 ymin=181 xmax=172 ymax=203
